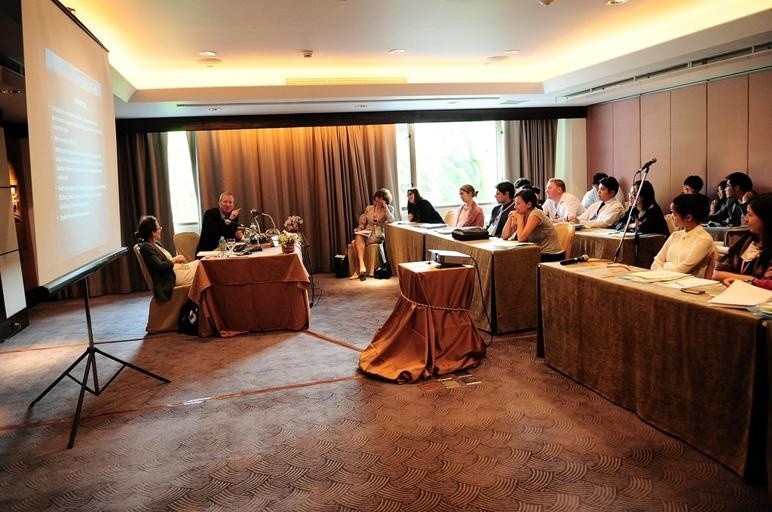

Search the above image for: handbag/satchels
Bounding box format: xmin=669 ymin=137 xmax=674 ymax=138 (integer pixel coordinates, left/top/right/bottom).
xmin=375 ymin=262 xmax=391 ymax=279
xmin=178 ymin=300 xmax=199 ymax=334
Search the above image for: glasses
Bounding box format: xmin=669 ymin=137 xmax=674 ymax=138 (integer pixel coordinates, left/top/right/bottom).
xmin=628 ymin=193 xmax=636 ymax=198
xmin=156 ymin=227 xmax=163 ymax=230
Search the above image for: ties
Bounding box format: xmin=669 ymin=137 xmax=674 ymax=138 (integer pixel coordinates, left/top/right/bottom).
xmin=597 ymin=202 xmax=605 ymax=215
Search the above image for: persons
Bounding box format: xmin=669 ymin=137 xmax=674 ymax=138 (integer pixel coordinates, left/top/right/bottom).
xmin=701 ymin=172 xmax=759 ymax=228
xmin=484 ymin=172 xmax=625 ymax=263
xmin=135 ymin=215 xmax=200 ymax=302
xmin=194 ymin=191 xmax=246 ymax=260
xmin=650 ymin=194 xmax=719 ymax=280
xmin=608 ymin=180 xmax=671 ymax=241
xmin=711 ymin=194 xmax=772 ymax=291
xmin=348 ymin=188 xmax=395 ymax=280
xmin=453 ymin=184 xmax=484 ymax=228
xmin=680 ymin=175 xmax=704 ymax=196
xmin=407 ymin=188 xmax=446 ymax=224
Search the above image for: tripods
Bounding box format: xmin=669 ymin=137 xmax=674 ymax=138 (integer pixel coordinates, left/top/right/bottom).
xmin=30 ymin=277 xmax=171 ymax=449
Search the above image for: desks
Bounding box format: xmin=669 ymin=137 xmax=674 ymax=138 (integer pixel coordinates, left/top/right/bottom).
xmin=534 ymin=258 xmax=771 ymax=488
xmin=188 ymin=233 xmax=311 ymax=338
xmin=574 ymin=226 xmax=665 ymax=273
xmin=386 ymin=257 xmax=489 ymax=383
xmin=388 ymin=221 xmax=449 ymax=277
xmin=425 ymin=227 xmax=542 ymax=337
xmin=700 ymin=226 xmax=753 ymax=261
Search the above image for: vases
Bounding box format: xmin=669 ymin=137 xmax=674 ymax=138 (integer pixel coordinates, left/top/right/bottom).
xmin=288 ymin=230 xmax=298 ymax=233
xmin=282 ymin=242 xmax=295 ymax=254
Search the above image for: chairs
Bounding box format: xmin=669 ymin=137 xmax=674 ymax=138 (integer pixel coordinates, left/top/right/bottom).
xmin=172 ymin=231 xmax=200 ymax=262
xmin=553 ymin=222 xmax=575 ymax=258
xmin=664 ymin=214 xmax=681 ymax=234
xmin=348 ymin=205 xmax=395 ymax=277
xmin=444 ymin=211 xmax=457 ymax=226
xmin=704 ymin=248 xmax=717 ymax=279
xmin=134 ymin=243 xmax=193 ymax=334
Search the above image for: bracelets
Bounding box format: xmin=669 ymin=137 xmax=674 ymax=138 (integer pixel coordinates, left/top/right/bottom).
xmin=226 ymin=217 xmax=232 ymax=222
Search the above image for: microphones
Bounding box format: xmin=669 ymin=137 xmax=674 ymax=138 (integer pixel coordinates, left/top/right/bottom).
xmin=560 ymin=254 xmax=589 ymax=265
xmin=249 ymin=208 xmax=264 ymax=216
xmin=638 ymin=158 xmax=657 ymax=173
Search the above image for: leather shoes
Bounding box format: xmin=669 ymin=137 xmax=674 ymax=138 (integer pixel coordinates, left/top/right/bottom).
xmin=349 ymin=272 xmax=364 ymax=279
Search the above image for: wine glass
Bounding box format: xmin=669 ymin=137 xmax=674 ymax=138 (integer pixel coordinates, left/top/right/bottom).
xmin=226 ymin=239 xmax=236 ymax=255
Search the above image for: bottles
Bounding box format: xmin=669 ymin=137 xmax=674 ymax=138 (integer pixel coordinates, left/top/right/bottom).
xmin=249 ymin=223 xmax=256 ymax=231
xmin=219 ymin=236 xmax=226 ymax=251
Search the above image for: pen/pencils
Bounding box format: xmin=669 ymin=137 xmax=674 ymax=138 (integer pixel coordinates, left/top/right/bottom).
xmin=175 ymin=249 xmax=178 ymax=257
xmin=609 ymin=231 xmax=620 ymax=235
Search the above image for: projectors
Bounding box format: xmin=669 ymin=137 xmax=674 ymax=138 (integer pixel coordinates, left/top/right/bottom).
xmin=428 ymin=249 xmax=471 ymax=264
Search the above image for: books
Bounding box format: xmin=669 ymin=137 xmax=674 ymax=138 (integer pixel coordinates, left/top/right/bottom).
xmin=354 ymin=230 xmax=372 ymax=238
xmin=414 ymin=223 xmax=448 ymax=229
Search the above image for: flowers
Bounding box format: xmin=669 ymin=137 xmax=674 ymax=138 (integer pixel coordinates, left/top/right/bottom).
xmin=278 ymin=234 xmax=297 ymax=245
xmin=285 ymin=216 xmax=303 ymax=231
